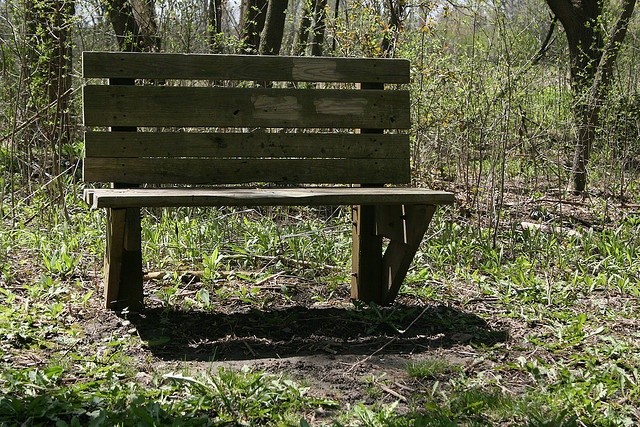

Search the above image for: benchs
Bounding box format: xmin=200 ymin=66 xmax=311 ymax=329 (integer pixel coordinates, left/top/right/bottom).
xmin=80 ymin=48 xmax=455 ymax=312
